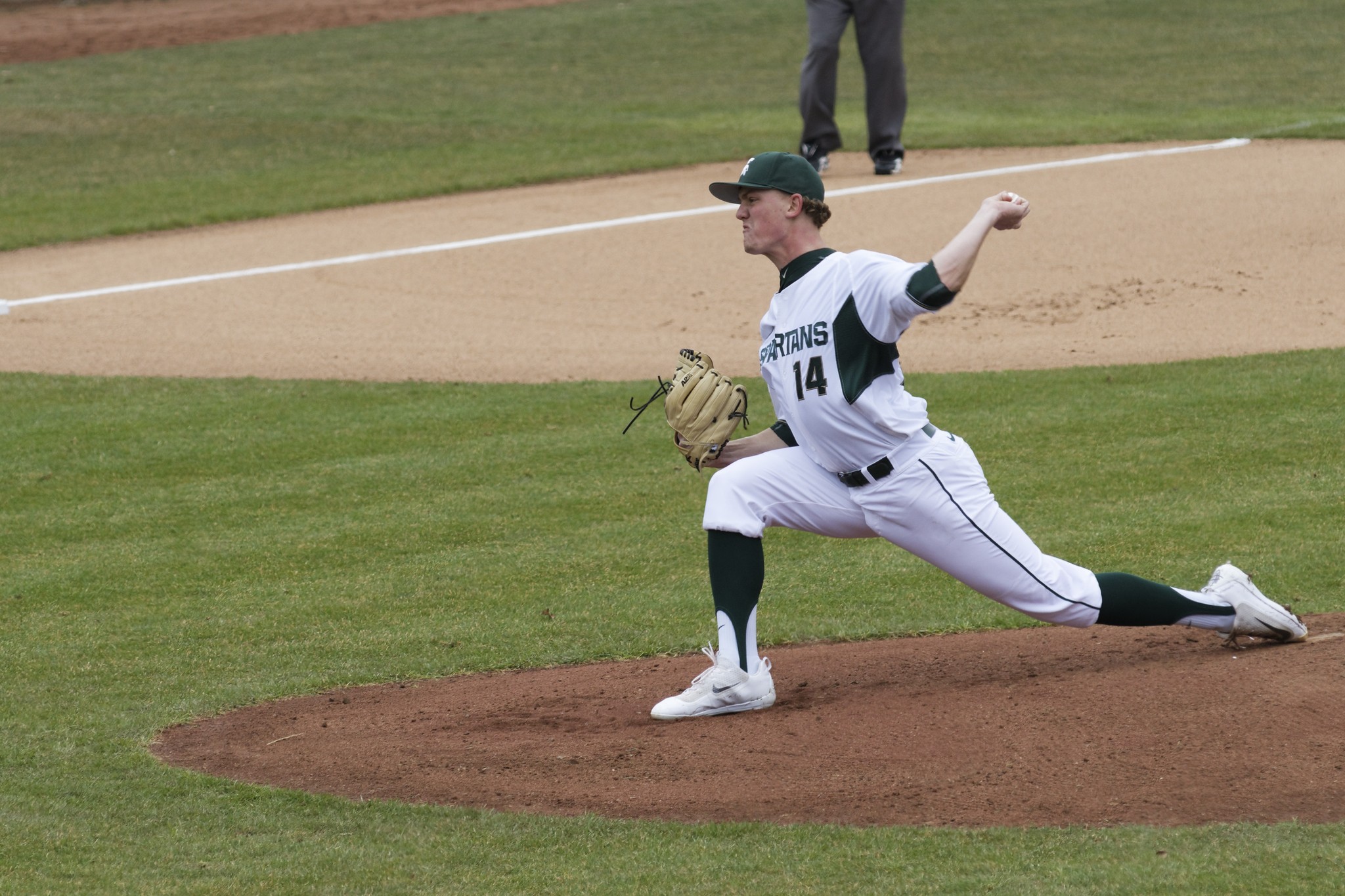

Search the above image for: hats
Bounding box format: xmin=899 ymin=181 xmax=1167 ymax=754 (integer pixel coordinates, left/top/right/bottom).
xmin=709 ymin=151 xmax=825 ymax=206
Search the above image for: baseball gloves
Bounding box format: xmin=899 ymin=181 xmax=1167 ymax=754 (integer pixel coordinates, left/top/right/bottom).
xmin=664 ymin=348 xmax=749 ymax=473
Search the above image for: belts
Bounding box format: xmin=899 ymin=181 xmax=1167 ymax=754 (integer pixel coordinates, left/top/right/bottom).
xmin=838 ymin=423 xmax=936 ymax=488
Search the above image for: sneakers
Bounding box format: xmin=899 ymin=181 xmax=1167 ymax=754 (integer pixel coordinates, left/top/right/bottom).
xmin=1200 ymin=561 xmax=1308 ymax=651
xmin=799 ymin=142 xmax=830 ymax=175
xmin=650 ymin=640 xmax=777 ymax=720
xmin=873 ymin=151 xmax=902 ymax=175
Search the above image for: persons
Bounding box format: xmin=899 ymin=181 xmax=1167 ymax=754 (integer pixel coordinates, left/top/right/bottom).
xmin=797 ymin=1 xmax=908 ymax=179
xmin=649 ymin=151 xmax=1309 ymax=721
